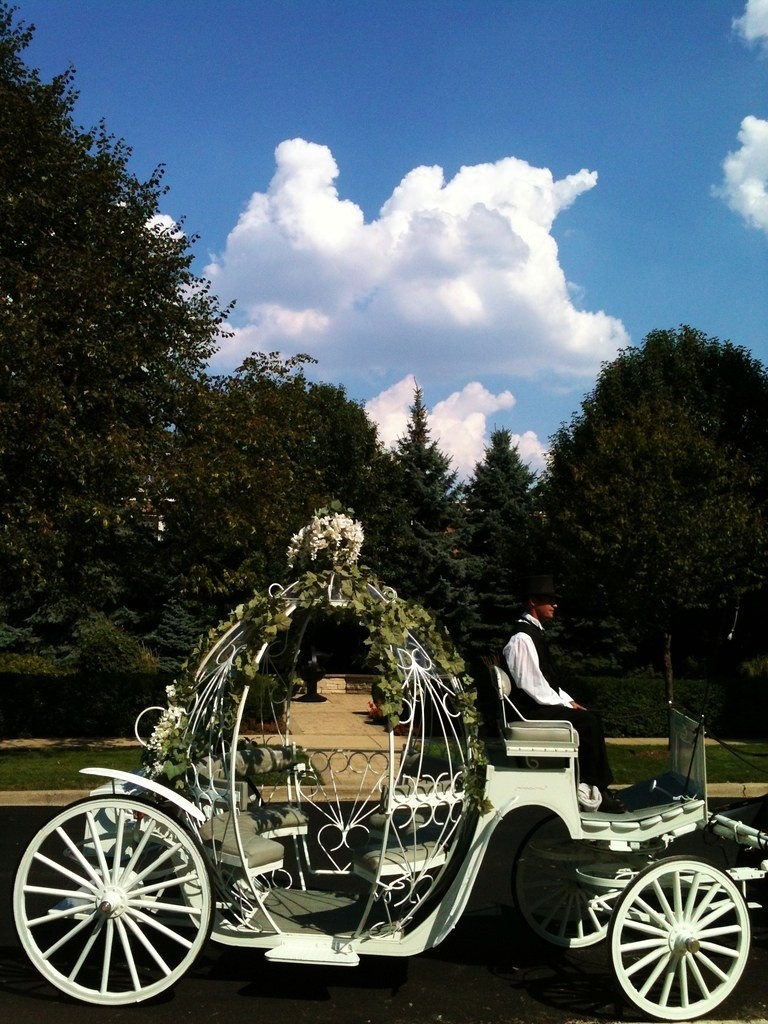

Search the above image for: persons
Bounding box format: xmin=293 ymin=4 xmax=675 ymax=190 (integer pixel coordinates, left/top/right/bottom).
xmin=499 ymin=578 xmax=626 ymax=812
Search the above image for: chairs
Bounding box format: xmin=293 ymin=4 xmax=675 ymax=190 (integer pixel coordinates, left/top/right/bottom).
xmin=466 ymin=646 xmax=583 ymax=813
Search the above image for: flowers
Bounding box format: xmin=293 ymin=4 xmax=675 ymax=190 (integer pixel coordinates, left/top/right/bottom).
xmin=146 ymin=679 xmax=184 ymax=774
xmin=286 ymin=500 xmax=364 ymax=573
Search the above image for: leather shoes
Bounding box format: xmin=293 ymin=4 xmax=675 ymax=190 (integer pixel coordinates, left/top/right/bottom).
xmin=598 ymin=789 xmax=627 ymax=813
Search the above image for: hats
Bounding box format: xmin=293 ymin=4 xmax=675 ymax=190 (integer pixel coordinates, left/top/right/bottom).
xmin=515 ymin=572 xmax=563 ymax=599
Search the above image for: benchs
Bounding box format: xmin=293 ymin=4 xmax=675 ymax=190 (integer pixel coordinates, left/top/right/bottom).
xmin=183 ymin=745 xmax=309 ymax=928
xmin=353 ymin=743 xmax=465 ymax=932
